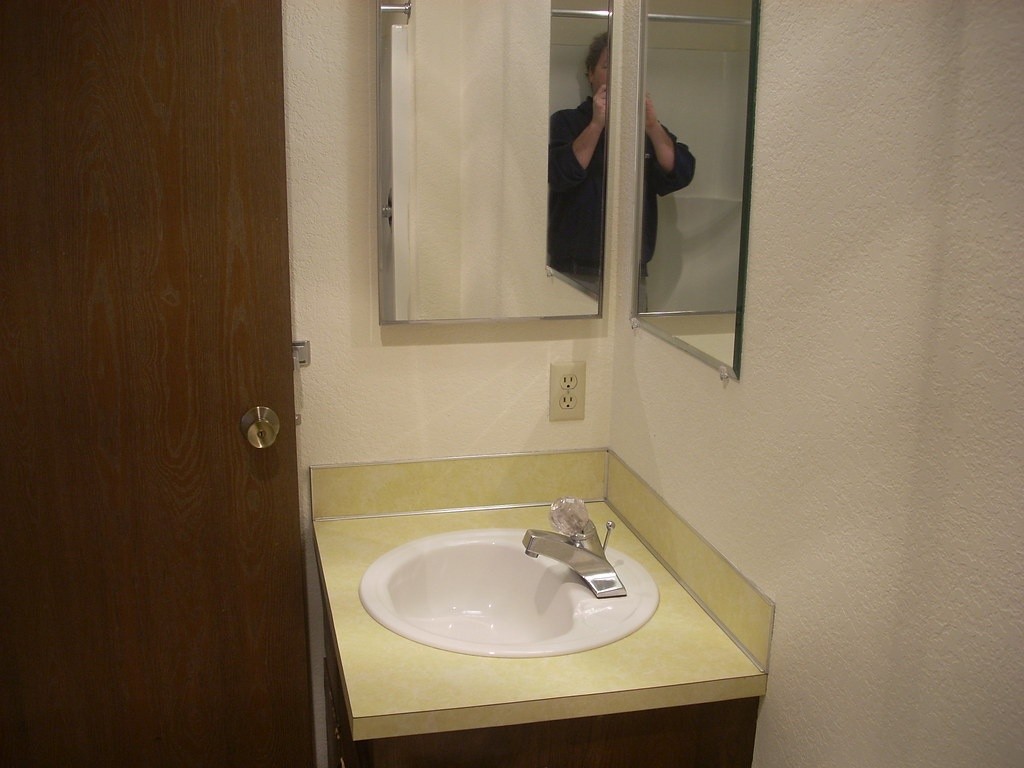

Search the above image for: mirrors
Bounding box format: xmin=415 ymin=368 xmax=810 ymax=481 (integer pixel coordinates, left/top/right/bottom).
xmin=630 ymin=0 xmax=760 ymax=380
xmin=376 ymin=0 xmax=618 ymax=325
xmin=546 ymin=43 xmax=611 ymax=301
xmin=639 ymin=49 xmax=750 ymax=317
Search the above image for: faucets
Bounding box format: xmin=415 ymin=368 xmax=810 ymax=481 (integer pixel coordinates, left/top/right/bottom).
xmin=521 ymin=497 xmax=628 ymax=599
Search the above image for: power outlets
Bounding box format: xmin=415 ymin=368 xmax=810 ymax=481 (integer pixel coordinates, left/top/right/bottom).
xmin=549 ymin=361 xmax=586 ymax=421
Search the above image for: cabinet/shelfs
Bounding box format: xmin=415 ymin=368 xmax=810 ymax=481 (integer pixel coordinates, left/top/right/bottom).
xmin=329 ymin=592 xmax=757 ymax=768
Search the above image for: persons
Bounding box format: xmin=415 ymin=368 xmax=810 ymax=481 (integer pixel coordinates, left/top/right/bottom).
xmin=545 ymin=34 xmax=697 ymax=313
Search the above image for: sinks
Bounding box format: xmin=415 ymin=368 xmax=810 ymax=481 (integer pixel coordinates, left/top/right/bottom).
xmin=357 ymin=527 xmax=659 ymax=658
